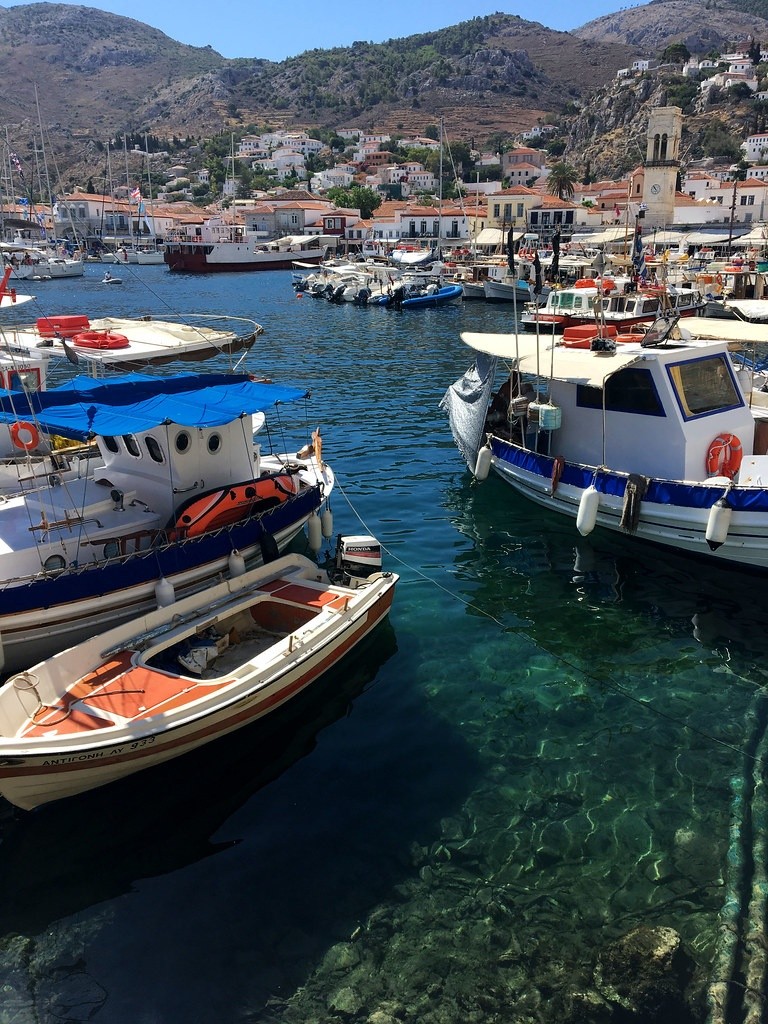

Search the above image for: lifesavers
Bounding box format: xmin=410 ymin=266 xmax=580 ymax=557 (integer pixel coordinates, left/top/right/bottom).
xmin=10 ymin=421 xmax=39 ymax=450
xmin=715 ymin=273 xmax=722 ymax=284
xmin=72 ymin=331 xmax=130 ymax=350
xmin=705 ymin=432 xmax=743 ymax=479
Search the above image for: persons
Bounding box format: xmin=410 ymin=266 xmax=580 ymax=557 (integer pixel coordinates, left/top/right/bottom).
xmin=25 ymin=252 xmax=30 ymax=259
xmin=105 ymin=271 xmax=111 ymax=280
xmin=426 ymin=284 xmax=442 ymax=296
xmin=11 ymin=254 xmax=17 ymax=264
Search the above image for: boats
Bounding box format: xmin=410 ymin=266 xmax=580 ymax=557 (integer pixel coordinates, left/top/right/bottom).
xmin=0 ymin=311 xmax=264 ymax=365
xmin=293 ymin=219 xmax=767 ymax=455
xmin=0 ymin=409 xmax=267 ymax=502
xmin=161 ymin=237 xmax=329 ymax=275
xmin=0 ymin=365 xmax=273 ymax=418
xmin=0 ymin=379 xmax=335 ymax=683
xmin=0 ymin=554 xmax=400 ymax=813
xmin=100 ymin=278 xmax=123 ymax=284
xmin=438 ymin=226 xmax=767 ymax=575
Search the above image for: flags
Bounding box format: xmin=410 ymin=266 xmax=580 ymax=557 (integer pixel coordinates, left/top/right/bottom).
xmin=631 ymin=225 xmax=647 ymax=282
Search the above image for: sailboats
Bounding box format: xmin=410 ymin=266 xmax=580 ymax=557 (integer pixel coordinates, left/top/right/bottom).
xmin=0 ymin=82 xmax=242 ymax=280
